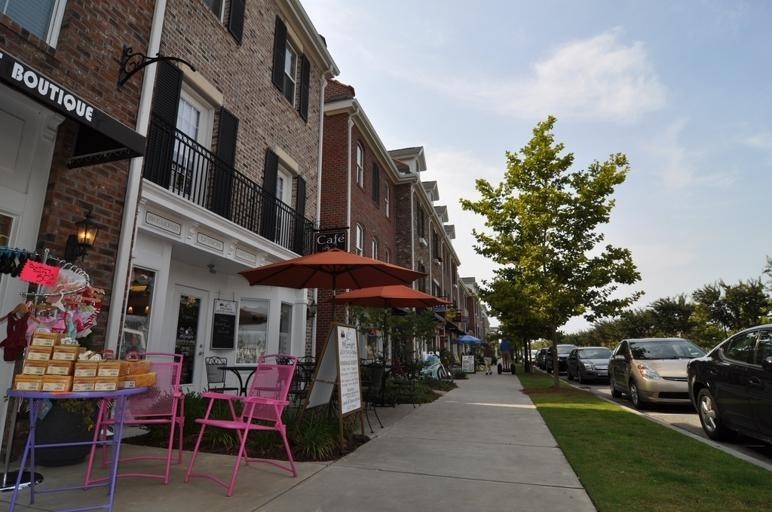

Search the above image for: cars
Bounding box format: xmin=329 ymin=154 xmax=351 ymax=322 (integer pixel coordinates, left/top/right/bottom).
xmin=528 ymin=324 xmax=772 ymax=445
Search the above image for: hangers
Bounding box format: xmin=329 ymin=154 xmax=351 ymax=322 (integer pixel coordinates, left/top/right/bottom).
xmin=0 ymin=246 xmax=105 ymax=324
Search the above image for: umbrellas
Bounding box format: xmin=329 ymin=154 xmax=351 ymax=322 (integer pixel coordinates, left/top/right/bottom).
xmin=237 ymin=247 xmax=429 ymax=326
xmin=331 ymin=285 xmax=451 ymax=409
xmin=454 ymin=335 xmax=483 ymax=345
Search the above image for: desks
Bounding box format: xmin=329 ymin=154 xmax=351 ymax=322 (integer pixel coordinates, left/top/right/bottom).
xmin=7 ymin=387 xmax=148 ymax=512
xmin=218 ymin=366 xmax=257 ymax=397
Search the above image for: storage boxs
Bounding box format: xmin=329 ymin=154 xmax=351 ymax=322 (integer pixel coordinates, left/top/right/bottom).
xmin=13 ymin=331 xmax=156 ymax=392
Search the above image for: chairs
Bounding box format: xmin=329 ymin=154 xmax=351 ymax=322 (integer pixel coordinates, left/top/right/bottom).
xmin=204 ymin=356 xmax=245 ymax=397
xmin=276 ymin=355 xmax=319 ymax=408
xmin=179 ymin=354 xmax=297 ymax=498
xmin=82 ymin=352 xmax=185 ymax=491
xmin=359 ymin=357 xmax=419 ymax=434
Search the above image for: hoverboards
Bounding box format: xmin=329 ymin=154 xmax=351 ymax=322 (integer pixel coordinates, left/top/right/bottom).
xmin=498 ymin=358 xmax=515 ymax=374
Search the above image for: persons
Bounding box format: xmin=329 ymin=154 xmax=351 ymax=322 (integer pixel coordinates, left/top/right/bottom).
xmin=484 ymin=345 xmax=493 ymax=375
xmin=499 ymin=336 xmax=512 ymax=371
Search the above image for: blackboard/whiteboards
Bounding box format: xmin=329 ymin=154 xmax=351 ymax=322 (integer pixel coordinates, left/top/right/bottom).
xmin=210 ymin=312 xmax=238 ymax=349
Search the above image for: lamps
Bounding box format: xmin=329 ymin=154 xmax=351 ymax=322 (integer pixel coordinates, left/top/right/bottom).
xmin=65 ymin=205 xmax=100 ymax=264
